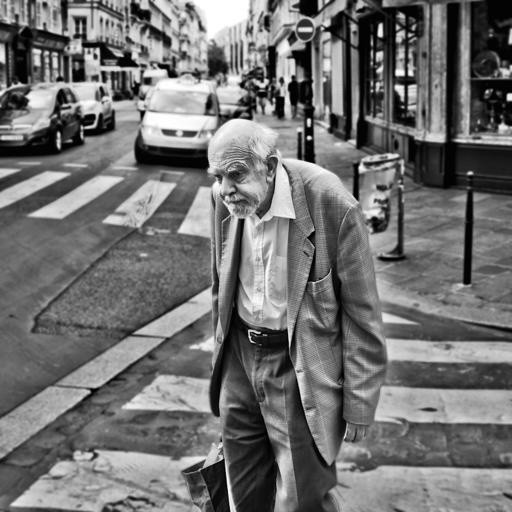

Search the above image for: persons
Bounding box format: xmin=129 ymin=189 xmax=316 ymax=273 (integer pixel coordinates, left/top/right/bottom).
xmin=276 ymin=77 xmax=286 ymax=119
xmin=288 ymin=75 xmax=299 ymax=119
xmin=206 ymin=116 xmax=389 ymax=512
xmin=11 ymin=75 xmax=22 ymax=86
xmin=56 ymin=76 xmax=64 ymax=82
xmin=192 ymin=67 xmax=201 ymax=82
xmin=244 ymin=72 xmax=275 ymax=114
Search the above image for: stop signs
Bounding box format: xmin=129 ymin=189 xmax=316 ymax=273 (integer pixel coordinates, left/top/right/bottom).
xmin=295 ymin=17 xmax=316 ymax=43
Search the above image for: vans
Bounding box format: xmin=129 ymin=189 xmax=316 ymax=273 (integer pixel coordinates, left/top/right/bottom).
xmin=138 ymin=69 xmax=168 ymax=100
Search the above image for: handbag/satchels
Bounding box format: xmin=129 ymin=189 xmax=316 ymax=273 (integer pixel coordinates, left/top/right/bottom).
xmin=181 ymin=445 xmax=230 ymax=512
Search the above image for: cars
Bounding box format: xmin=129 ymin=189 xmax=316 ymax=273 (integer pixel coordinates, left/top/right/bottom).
xmin=135 ymin=74 xmax=253 ymax=167
xmin=0 ymin=83 xmax=84 ymax=154
xmin=64 ymin=81 xmax=115 ymax=135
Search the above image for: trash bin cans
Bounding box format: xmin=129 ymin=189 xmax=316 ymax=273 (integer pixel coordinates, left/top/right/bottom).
xmin=357 ymin=153 xmax=400 ymax=234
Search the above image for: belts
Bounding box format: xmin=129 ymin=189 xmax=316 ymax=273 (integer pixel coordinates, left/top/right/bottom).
xmin=240 ymin=325 xmax=285 ymax=348
